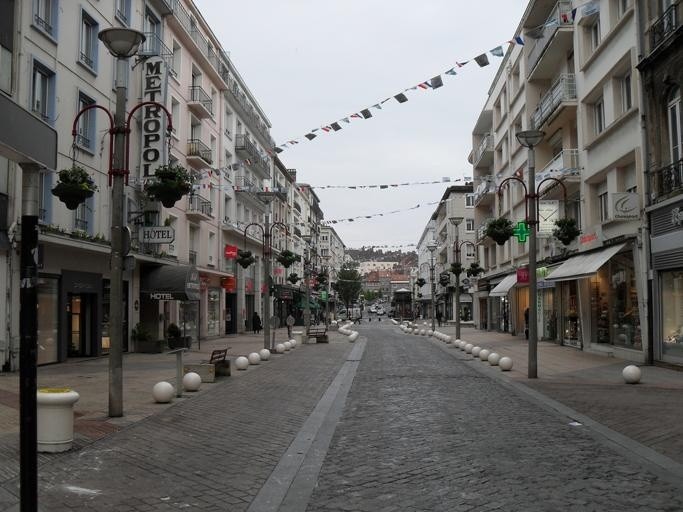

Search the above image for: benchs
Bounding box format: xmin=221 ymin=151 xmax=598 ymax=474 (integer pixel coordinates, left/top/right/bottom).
xmin=183 ymin=346 xmax=233 ymax=383
xmin=302 ymin=327 xmax=330 ymax=345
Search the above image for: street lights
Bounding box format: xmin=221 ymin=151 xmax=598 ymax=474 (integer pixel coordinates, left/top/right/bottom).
xmin=486 ymin=129 xmax=582 ymax=379
xmin=416 ymin=216 xmax=486 ymax=339
xmin=49 ymin=27 xmax=193 ymax=418
xmin=235 ymin=193 xmax=339 ymax=351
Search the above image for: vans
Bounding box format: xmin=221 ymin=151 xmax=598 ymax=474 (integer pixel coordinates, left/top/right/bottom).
xmin=335 ymin=308 xmax=362 ymax=324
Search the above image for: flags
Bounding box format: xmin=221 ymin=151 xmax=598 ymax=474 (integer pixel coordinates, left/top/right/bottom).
xmin=185 ymin=6 xmax=581 ymax=190
xmin=222 ymin=191 xmax=479 ymax=227
xmin=360 ymin=244 xmax=416 ymax=250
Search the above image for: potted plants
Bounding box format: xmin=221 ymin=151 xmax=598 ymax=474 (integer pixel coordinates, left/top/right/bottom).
xmin=233 ymin=246 xmax=302 ymax=285
xmin=415 ymin=260 xmax=487 ymax=289
xmin=130 ymin=322 xmax=192 ymax=353
xmin=50 ymin=163 xmax=101 ymax=210
xmin=483 ymin=217 xmax=585 ymax=246
xmin=141 ymin=159 xmax=198 ymax=209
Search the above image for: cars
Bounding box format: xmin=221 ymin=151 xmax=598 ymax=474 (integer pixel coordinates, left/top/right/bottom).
xmin=368 ymin=302 xmax=387 ymax=315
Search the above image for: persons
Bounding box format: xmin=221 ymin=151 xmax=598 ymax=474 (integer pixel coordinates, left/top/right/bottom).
xmin=436 ymin=310 xmax=441 ymax=327
xmin=252 ymin=312 xmax=261 ymax=334
xmin=285 ymin=314 xmax=295 ymax=337
xmin=524 ymin=308 xmax=529 ymax=339
xmin=353 ymin=309 xmax=361 ymax=325
